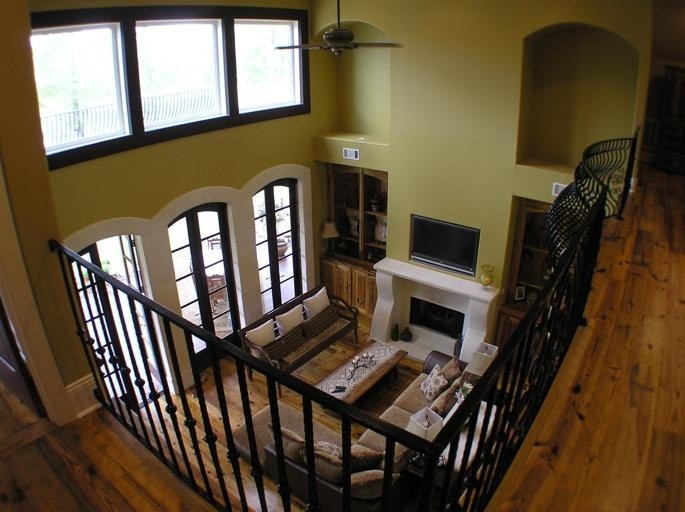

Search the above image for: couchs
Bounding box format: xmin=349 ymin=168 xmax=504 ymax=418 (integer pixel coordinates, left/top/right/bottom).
xmin=228 ymin=350 xmax=495 ymax=510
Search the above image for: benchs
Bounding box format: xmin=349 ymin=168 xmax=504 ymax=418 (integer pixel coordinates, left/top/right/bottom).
xmin=231 ymin=283 xmax=360 ymax=396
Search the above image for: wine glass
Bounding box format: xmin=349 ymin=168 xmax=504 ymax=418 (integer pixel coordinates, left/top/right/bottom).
xmin=479 ymin=265 xmax=495 ymax=290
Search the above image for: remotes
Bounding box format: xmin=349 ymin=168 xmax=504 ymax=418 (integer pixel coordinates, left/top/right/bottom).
xmin=332 ymin=389 xmax=345 ymax=392
xmin=336 ymin=386 xmax=346 ymax=389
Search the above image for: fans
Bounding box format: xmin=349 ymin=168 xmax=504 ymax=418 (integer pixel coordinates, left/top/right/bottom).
xmin=275 ymin=1 xmax=402 ymax=56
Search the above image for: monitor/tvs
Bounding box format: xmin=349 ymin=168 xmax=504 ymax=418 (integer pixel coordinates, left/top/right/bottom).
xmin=409 ymin=214 xmax=481 ymax=277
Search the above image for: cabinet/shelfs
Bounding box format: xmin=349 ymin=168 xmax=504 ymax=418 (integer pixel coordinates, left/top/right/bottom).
xmin=494 ymin=195 xmax=587 ymax=384
xmin=321 ymin=162 xmax=388 ymax=319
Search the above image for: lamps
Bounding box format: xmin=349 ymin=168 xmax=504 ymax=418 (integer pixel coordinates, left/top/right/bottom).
xmin=319 ymin=222 xmax=340 ymax=258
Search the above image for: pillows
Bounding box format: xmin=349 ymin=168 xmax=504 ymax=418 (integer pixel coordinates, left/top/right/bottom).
xmin=421 ymin=357 xmax=464 ymax=415
xmin=244 ymin=286 xmax=337 ymax=361
xmin=265 ymin=420 xmax=410 ymax=500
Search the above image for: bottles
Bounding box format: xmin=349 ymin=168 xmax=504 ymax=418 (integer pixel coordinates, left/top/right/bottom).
xmin=391 ymin=323 xmax=412 ymax=342
